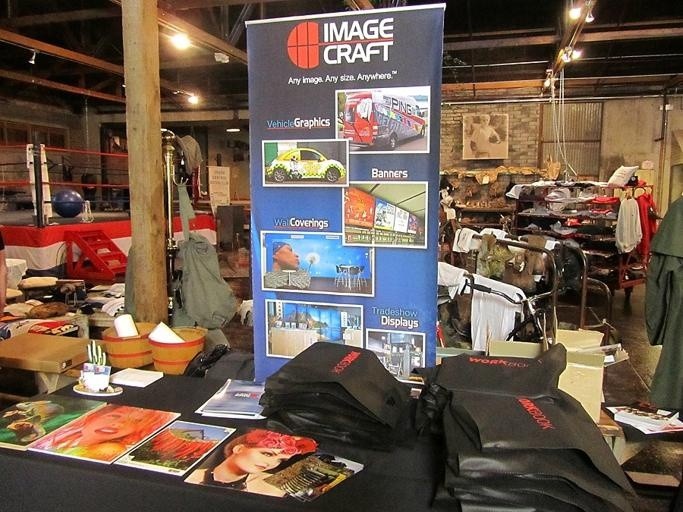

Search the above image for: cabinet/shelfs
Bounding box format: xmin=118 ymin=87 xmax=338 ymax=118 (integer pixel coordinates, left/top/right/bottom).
xmin=441 ymin=170 xmax=633 ymax=309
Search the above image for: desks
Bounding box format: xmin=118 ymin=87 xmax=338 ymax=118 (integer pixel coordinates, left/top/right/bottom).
xmin=1 ymin=366 xmax=447 ymax=510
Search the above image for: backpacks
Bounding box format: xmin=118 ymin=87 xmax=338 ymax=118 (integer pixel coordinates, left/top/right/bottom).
xmin=258 ymin=340 xmax=413 ymax=452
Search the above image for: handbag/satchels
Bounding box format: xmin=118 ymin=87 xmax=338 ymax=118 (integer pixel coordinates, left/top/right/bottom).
xmin=177 ymin=183 xmax=237 ymax=330
xmin=171 ymin=288 xmax=232 ymax=353
xmin=410 ymin=344 xmax=638 ymax=511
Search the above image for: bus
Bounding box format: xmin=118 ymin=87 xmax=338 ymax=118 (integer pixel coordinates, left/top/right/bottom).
xmin=342 ymin=90 xmax=427 ymax=150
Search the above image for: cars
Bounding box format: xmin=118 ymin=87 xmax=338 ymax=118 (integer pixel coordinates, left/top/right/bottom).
xmin=264 ymin=146 xmax=346 ymax=184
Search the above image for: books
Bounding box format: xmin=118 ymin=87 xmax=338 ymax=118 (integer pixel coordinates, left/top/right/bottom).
xmin=613 ymin=400 xmax=679 ymax=431
xmin=604 ymin=349 xmax=618 ymax=364
xmin=109 ymin=368 xmax=164 ymax=389
xmin=193 ymin=378 xmax=266 ymax=420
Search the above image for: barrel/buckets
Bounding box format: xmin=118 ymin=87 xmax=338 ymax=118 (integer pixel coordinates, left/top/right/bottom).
xmin=100 ymin=322 xmax=159 ymax=368
xmin=148 ymin=326 xmax=206 ymax=376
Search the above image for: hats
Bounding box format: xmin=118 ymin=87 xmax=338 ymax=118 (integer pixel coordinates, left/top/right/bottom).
xmin=273 ymin=240 xmax=289 ymax=256
xmin=175 ymin=134 xmax=204 ymax=175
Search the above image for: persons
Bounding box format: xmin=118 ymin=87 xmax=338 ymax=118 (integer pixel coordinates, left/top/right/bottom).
xmin=271 ymin=240 xmax=300 ymax=270
xmin=0 ymin=231 xmax=9 ymax=319
xmin=183 ymin=428 xmax=317 ymax=497
xmin=29 ymin=403 xmax=174 ymax=464
xmin=469 ymin=114 xmax=500 ymax=159
